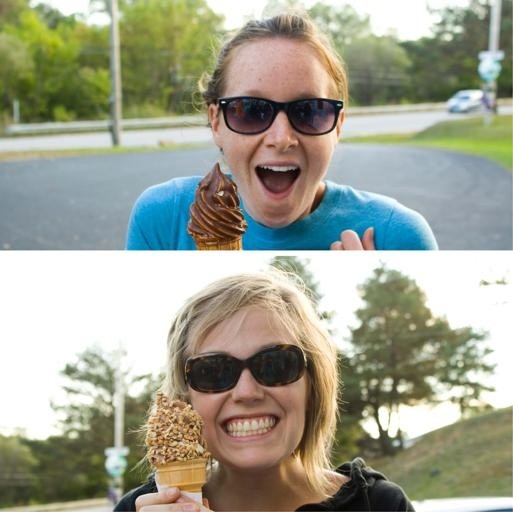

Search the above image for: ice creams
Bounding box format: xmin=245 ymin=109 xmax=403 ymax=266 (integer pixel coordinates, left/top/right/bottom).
xmin=144 ymin=392 xmax=212 ymax=505
xmin=186 ymin=162 xmax=248 ymax=250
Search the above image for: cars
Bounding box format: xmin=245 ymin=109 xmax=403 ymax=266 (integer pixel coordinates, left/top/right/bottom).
xmin=448 ymin=89 xmax=484 ymax=112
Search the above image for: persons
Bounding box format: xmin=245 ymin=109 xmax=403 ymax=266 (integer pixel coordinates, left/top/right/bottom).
xmin=124 ymin=12 xmax=440 ymax=251
xmin=113 ymin=269 xmax=414 ymax=512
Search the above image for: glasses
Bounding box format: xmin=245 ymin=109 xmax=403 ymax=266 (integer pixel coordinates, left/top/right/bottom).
xmin=184 ymin=344 xmax=310 ymax=395
xmin=213 ymin=95 xmax=343 ymax=136
